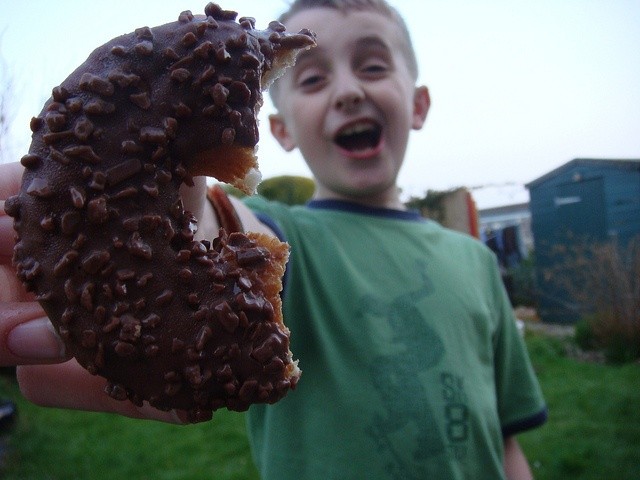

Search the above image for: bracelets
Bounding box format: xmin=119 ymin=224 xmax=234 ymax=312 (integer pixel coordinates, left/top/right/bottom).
xmin=206 ymin=183 xmax=248 ymax=236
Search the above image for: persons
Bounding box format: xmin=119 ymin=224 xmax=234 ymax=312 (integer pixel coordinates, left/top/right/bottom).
xmin=0 ymin=0 xmax=549 ymax=480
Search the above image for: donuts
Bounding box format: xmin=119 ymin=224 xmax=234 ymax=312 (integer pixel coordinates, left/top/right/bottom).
xmin=5 ymin=2 xmax=316 ymax=414
